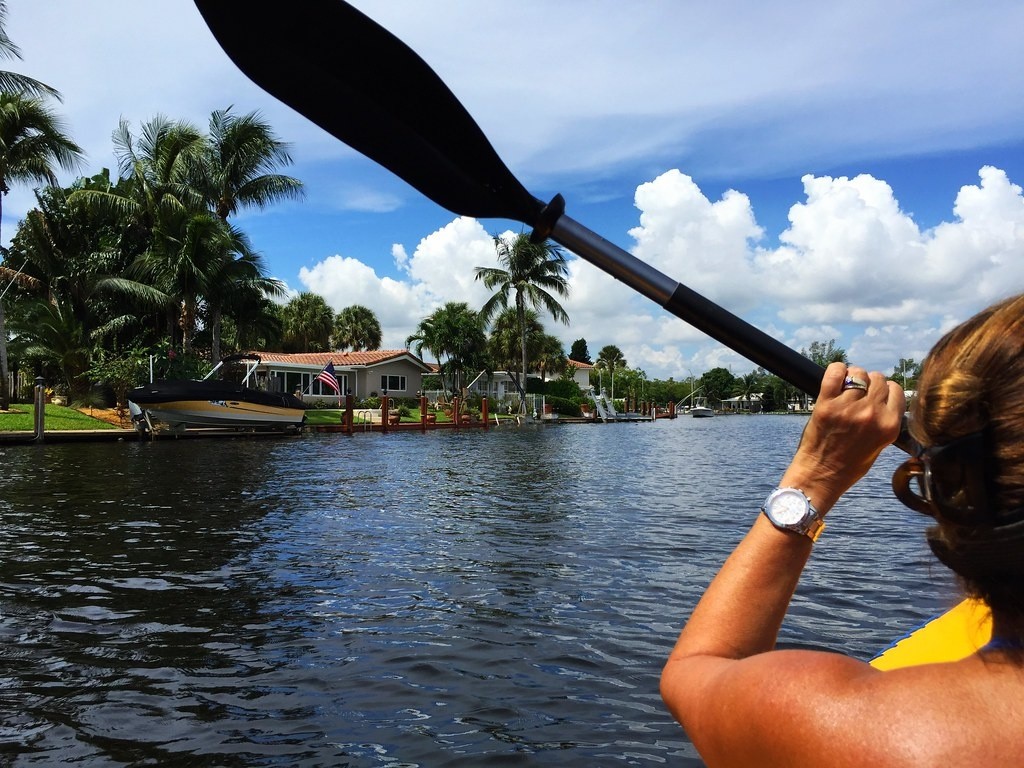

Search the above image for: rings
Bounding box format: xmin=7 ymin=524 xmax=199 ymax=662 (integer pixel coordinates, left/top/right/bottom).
xmin=843 ymin=376 xmax=867 ymax=391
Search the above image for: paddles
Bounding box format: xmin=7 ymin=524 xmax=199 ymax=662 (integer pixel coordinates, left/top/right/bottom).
xmin=189 ymin=0 xmax=928 ymax=461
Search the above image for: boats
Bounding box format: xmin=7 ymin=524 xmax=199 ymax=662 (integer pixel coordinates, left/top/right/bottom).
xmin=124 ymin=353 xmax=309 ymax=441
xmin=690 ymin=406 xmax=714 ymax=417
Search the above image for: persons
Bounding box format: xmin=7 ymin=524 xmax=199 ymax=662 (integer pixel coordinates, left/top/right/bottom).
xmin=662 ymin=294 xmax=1024 ymax=767
xmin=44 ymin=385 xmax=54 ymax=402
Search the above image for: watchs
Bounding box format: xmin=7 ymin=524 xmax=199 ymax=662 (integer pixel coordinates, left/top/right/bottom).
xmin=761 ymin=487 xmax=825 ymax=543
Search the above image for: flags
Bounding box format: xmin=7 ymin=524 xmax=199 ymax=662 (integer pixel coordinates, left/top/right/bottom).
xmin=317 ymin=361 xmax=342 ymax=407
